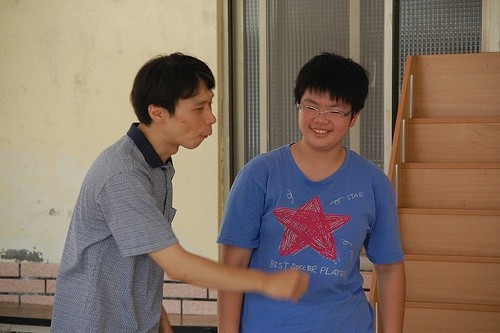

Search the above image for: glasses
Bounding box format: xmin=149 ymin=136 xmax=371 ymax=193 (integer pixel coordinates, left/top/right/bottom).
xmin=296 ymin=100 xmax=353 ymax=120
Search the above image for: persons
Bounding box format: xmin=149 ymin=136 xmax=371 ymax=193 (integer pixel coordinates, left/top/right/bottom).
xmin=217 ymin=52 xmax=406 ymax=333
xmin=50 ymin=53 xmax=309 ymax=333
